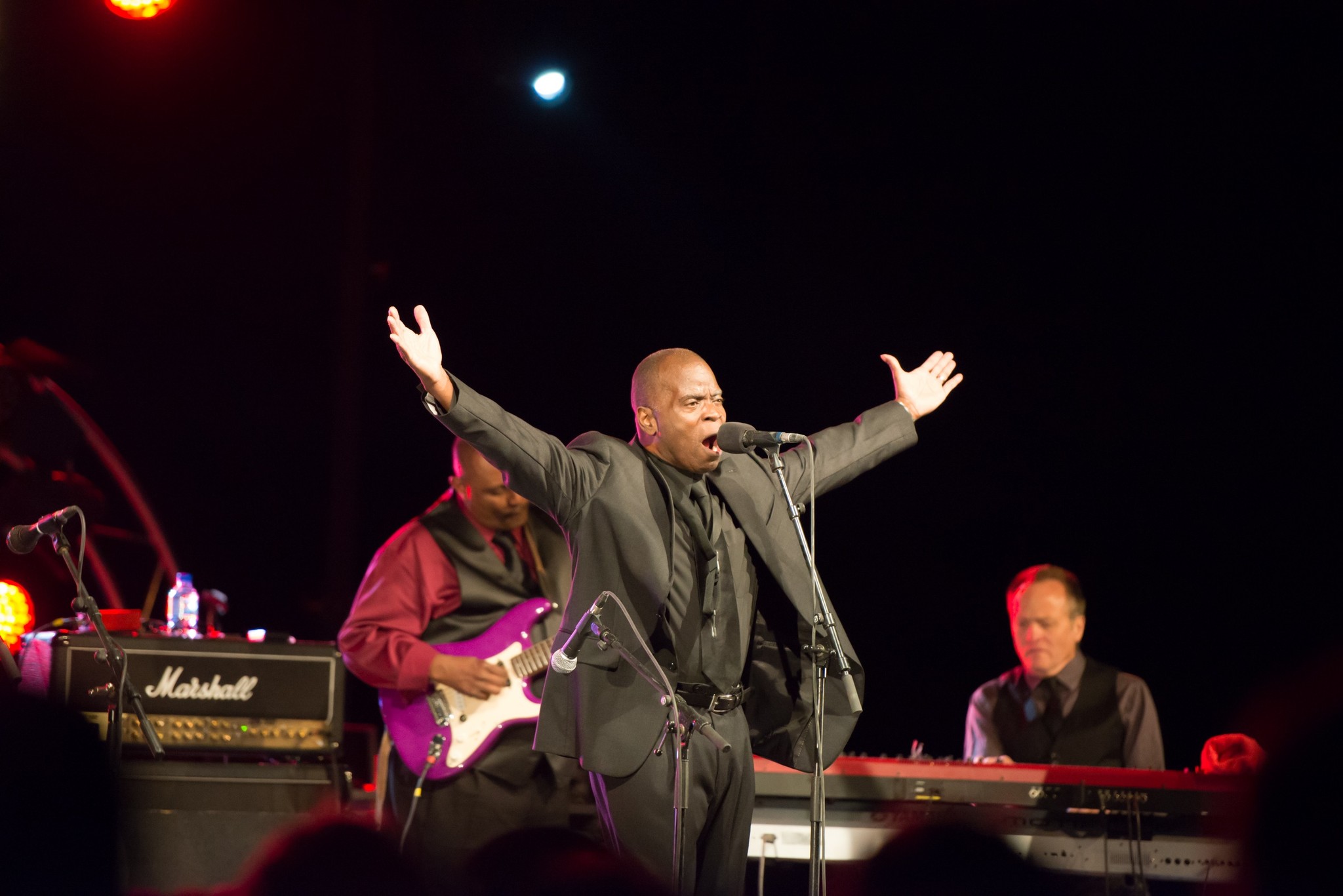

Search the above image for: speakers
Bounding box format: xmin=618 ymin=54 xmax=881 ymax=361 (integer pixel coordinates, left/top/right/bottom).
xmin=113 ymin=757 xmax=340 ymax=896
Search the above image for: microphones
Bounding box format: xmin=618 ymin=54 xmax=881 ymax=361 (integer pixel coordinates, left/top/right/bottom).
xmin=551 ymin=591 xmax=608 ymax=674
xmin=717 ymin=422 xmax=807 ymax=455
xmin=6 ymin=506 xmax=76 ymax=555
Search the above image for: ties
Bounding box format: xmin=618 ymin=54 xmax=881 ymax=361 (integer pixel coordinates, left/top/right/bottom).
xmin=691 ymin=478 xmax=712 ymax=538
xmin=1039 ymin=677 xmax=1063 ymax=743
xmin=493 ymin=530 xmax=533 ymax=592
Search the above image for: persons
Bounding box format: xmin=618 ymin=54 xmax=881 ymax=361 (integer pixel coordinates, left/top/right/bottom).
xmin=965 ymin=564 xmax=1166 ymax=770
xmin=338 ymin=435 xmax=572 ymax=856
xmin=388 ymin=304 xmax=964 ymax=895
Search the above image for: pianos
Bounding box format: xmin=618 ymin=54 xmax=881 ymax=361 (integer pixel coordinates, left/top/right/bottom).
xmin=747 ymin=755 xmax=1256 ymax=894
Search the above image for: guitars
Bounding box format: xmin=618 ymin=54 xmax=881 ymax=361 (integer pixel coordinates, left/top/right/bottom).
xmin=373 ymin=594 xmax=558 ymax=783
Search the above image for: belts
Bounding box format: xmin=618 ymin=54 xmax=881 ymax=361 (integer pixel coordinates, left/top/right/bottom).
xmin=679 ymin=685 xmax=751 ymax=714
